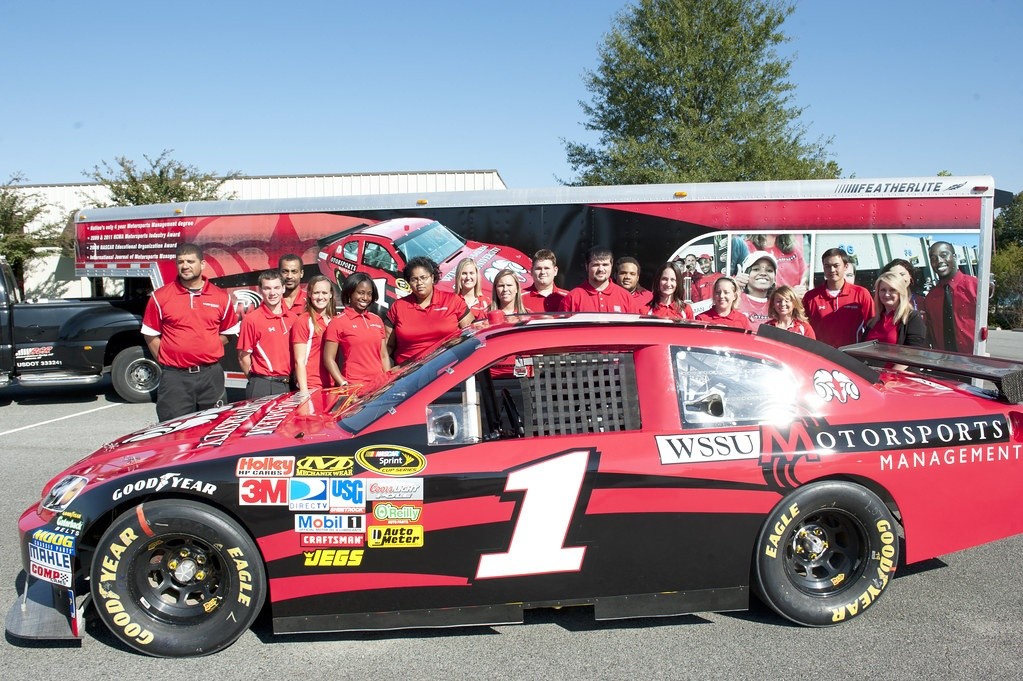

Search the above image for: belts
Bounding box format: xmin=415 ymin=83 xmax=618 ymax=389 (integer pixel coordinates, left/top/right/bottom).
xmin=249 ymin=372 xmax=289 ymax=383
xmin=160 ymin=361 xmax=220 ymax=373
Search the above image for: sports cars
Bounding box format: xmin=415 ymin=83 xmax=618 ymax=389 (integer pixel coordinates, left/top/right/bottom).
xmin=317 ymin=216 xmax=543 ymax=318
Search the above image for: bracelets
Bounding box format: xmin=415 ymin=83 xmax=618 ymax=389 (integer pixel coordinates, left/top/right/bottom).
xmin=340 ymin=381 xmax=348 ymax=386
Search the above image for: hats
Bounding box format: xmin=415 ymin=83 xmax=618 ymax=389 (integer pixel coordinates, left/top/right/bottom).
xmin=696 ymin=254 xmax=712 ymax=262
xmin=742 ymin=251 xmax=778 ymax=276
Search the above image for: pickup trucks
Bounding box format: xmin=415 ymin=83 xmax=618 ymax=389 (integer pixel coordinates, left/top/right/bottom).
xmin=0 ymin=256 xmax=165 ymax=406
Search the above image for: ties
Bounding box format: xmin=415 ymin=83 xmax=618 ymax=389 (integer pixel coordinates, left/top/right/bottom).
xmin=943 ymin=283 xmax=958 ymax=353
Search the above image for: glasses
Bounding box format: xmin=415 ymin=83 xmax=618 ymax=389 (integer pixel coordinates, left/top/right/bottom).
xmin=408 ymin=272 xmax=434 ymax=282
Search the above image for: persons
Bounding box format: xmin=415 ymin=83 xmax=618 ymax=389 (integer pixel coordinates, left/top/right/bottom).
xmin=558 ymin=246 xmax=639 ymax=314
xmin=383 ymin=256 xmax=474 ymax=364
xmin=291 ymin=276 xmax=337 ymax=390
xmin=612 ymin=257 xmax=651 ymax=307
xmin=478 ymin=269 xmax=534 ymax=377
xmin=881 ymin=242 xmax=980 ymax=353
xmin=638 ymin=262 xmax=694 ymax=319
xmin=141 ymin=242 xmax=237 ymax=424
xmin=455 ymin=258 xmax=490 ymax=322
xmin=695 ymin=277 xmax=752 ymax=330
xmin=804 ymin=248 xmax=875 ymax=348
xmin=237 ymin=269 xmax=300 ymax=400
xmin=764 ymin=285 xmax=816 ymax=341
xmin=675 ymin=233 xmax=809 ymax=302
xmin=277 ymin=254 xmax=308 ymax=318
xmin=822 ymin=253 xmax=856 ymax=284
xmin=324 ymin=272 xmax=390 ymax=387
xmin=521 ymin=248 xmax=569 ymax=313
xmin=862 ymin=272 xmax=928 ymax=372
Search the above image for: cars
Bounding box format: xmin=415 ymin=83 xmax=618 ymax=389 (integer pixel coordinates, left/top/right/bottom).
xmin=30 ymin=313 xmax=1023 ymax=657
